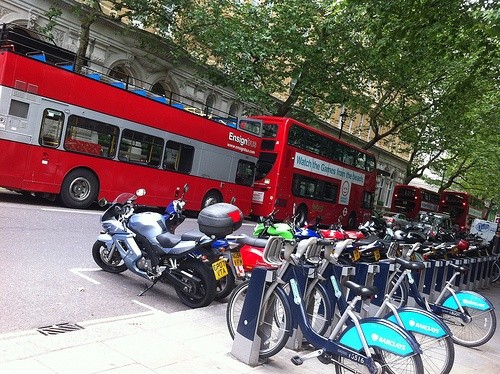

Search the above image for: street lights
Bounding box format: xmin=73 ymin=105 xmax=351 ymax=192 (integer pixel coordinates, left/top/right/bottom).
xmin=337 ymin=109 xmax=349 ymax=139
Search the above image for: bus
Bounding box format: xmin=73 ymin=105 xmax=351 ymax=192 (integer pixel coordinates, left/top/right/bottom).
xmin=247 ymin=116 xmax=378 ymax=230
xmin=389 ymin=186 xmax=439 ymax=223
xmin=441 ymin=191 xmax=485 ymax=233
xmin=0 ymin=22 xmax=263 ymax=218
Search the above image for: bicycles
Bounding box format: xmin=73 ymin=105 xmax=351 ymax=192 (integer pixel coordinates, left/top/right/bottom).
xmin=92 ymin=180 xmax=499 ymax=374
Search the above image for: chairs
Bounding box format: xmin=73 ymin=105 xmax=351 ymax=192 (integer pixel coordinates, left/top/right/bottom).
xmin=1 ymin=45 xmax=184 ymax=109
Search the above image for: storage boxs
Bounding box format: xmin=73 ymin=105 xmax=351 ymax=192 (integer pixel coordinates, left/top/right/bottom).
xmin=198 ymin=202 xmax=244 ymax=236
